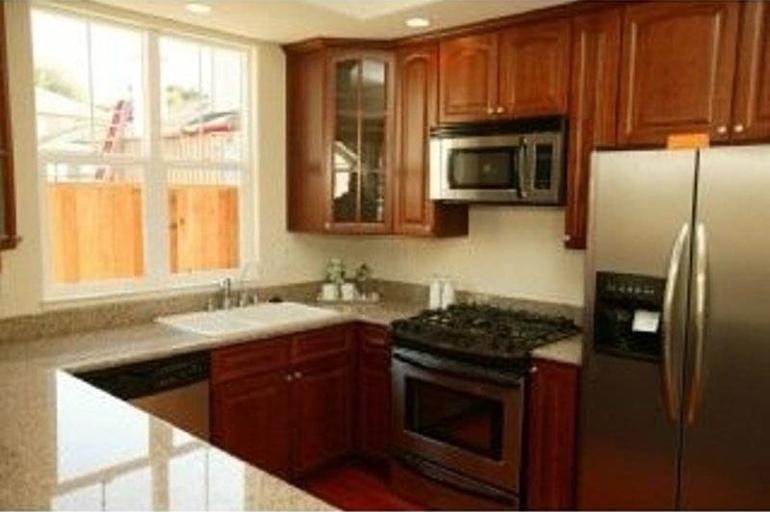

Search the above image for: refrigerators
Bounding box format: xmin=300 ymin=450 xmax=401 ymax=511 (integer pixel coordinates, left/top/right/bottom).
xmin=581 ymin=144 xmax=770 ymax=512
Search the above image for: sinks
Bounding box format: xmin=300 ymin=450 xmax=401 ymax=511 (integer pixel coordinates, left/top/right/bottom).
xmin=231 ymin=301 xmax=340 ymax=325
xmin=150 ymin=309 xmax=264 ymax=336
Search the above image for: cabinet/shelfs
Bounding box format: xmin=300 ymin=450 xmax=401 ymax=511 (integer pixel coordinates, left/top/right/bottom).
xmin=353 ymin=323 xmax=392 ymax=465
xmin=563 ymin=0 xmax=615 ymax=251
xmin=389 ymin=38 xmax=468 ymax=236
xmin=524 ymin=356 xmax=581 ymax=511
xmin=212 ymin=322 xmax=351 ymax=486
xmin=278 ymin=37 xmax=395 ymax=237
xmin=439 ymin=5 xmax=572 ymax=123
xmin=616 ymin=0 xmax=770 ymax=149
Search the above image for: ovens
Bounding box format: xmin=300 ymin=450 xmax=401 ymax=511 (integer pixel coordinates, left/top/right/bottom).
xmin=383 ymin=351 xmax=533 ymax=506
xmin=425 ymin=131 xmax=564 ymax=207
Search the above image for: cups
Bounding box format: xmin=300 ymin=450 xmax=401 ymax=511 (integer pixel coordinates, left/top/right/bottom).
xmin=321 ymin=283 xmax=353 ymax=300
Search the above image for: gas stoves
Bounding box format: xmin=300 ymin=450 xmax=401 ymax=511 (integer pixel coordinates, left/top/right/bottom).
xmin=388 ymin=302 xmax=576 ymax=364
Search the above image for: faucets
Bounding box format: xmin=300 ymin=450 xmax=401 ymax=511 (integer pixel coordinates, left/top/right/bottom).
xmin=219 ymin=277 xmax=233 ymax=311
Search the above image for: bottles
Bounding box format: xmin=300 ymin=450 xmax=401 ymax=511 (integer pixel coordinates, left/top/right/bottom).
xmin=441 ymin=280 xmax=456 ymax=307
xmin=428 ymin=276 xmax=440 ymax=310
